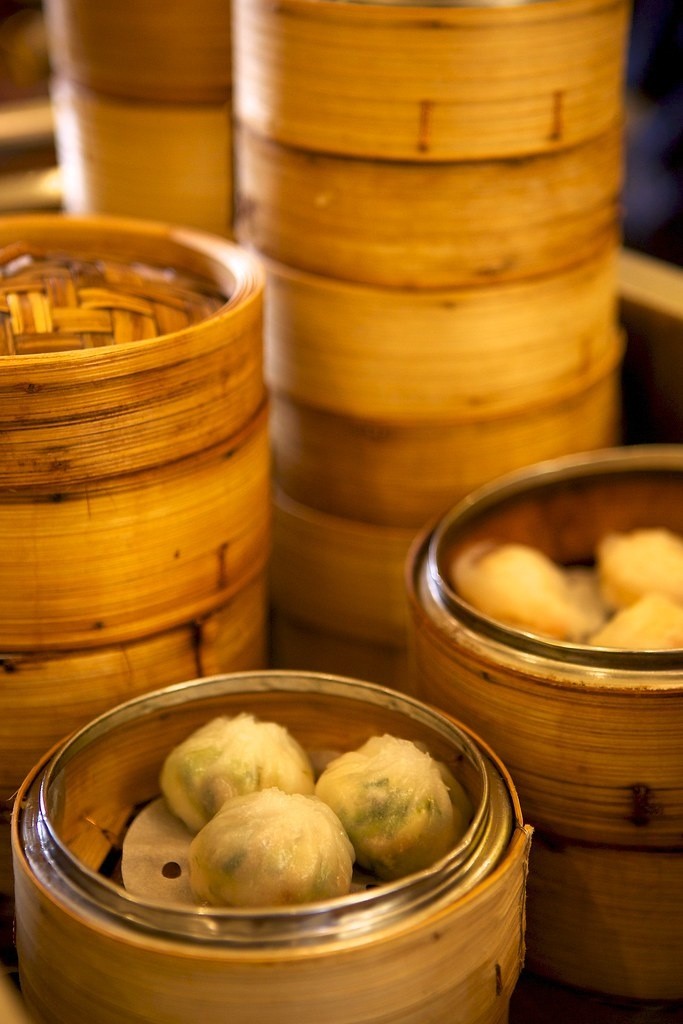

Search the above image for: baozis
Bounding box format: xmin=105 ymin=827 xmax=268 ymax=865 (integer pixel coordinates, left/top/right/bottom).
xmin=161 ymin=712 xmax=475 ymax=910
xmin=457 ymin=526 xmax=683 ymax=652
